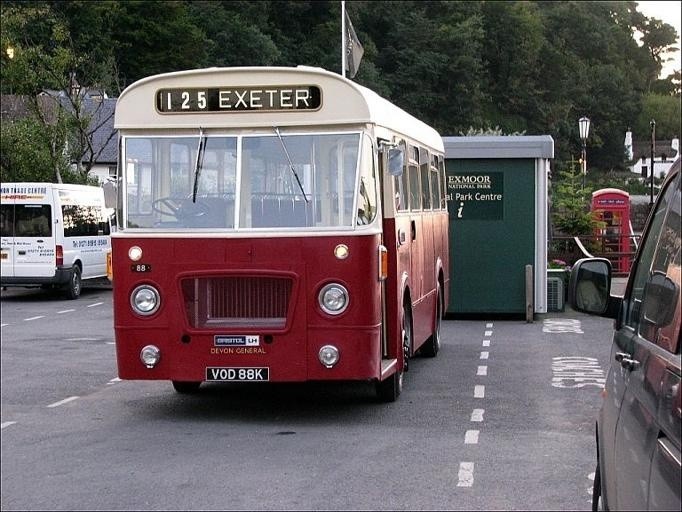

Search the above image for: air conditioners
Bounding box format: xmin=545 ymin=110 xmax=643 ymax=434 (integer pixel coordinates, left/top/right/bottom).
xmin=547 ymin=277 xmax=562 ymax=311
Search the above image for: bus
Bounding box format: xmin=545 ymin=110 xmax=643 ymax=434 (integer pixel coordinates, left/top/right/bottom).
xmin=109 ymin=64 xmax=450 ymax=404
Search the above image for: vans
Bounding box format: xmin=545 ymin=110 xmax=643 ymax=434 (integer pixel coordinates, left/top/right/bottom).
xmin=1 ymin=181 xmax=111 ymax=300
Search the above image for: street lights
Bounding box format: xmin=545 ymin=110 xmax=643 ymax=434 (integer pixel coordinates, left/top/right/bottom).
xmin=578 ymin=115 xmax=591 ymax=201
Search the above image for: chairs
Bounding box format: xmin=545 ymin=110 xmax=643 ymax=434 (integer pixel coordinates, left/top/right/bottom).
xmin=177 ymin=192 xmax=354 ymax=226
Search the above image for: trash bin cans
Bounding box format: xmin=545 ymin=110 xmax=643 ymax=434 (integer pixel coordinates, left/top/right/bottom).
xmin=547 ymin=269 xmax=566 ymax=312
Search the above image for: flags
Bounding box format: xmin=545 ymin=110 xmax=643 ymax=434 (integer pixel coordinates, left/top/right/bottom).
xmin=342 ymin=8 xmax=365 ymax=81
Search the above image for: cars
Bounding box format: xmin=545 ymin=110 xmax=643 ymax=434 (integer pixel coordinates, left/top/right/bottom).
xmin=567 ymin=157 xmax=682 ymax=512
